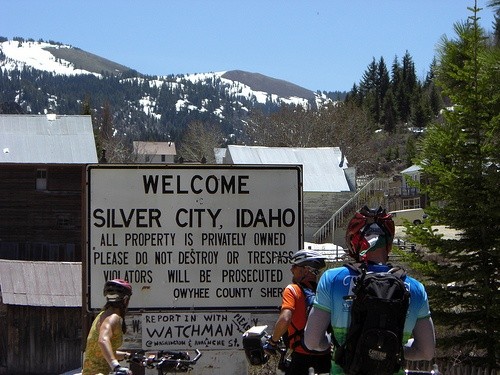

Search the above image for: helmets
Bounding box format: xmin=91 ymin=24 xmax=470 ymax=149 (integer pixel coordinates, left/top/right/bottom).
xmin=345 ymin=204 xmax=395 ymax=258
xmin=289 ymin=250 xmax=326 ymax=269
xmin=103 ymin=278 xmax=133 ymax=299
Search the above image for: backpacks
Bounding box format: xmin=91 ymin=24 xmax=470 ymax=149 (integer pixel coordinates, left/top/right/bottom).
xmin=295 ymin=281 xmax=334 ymax=355
xmin=329 ymin=260 xmax=413 ymax=375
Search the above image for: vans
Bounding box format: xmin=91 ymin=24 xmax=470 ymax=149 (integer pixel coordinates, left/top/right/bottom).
xmin=388 ymin=208 xmax=427 ymax=226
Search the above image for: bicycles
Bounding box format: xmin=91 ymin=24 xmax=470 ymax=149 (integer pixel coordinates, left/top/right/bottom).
xmin=243 ymin=324 xmax=292 ymax=375
xmin=110 ymin=346 xmax=202 ymax=375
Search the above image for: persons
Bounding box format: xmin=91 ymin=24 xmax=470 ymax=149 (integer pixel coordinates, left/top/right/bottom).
xmin=81 ymin=278 xmax=133 ymax=375
xmin=263 ymin=249 xmax=332 ymax=375
xmin=302 ymin=204 xmax=436 ymax=375
